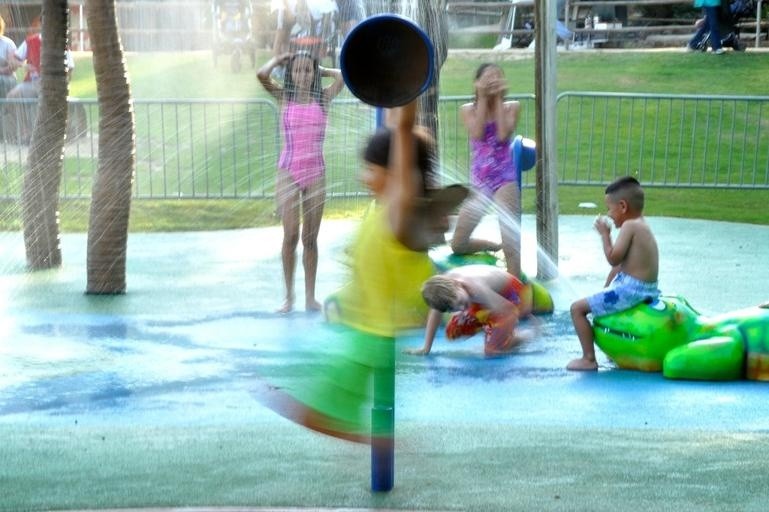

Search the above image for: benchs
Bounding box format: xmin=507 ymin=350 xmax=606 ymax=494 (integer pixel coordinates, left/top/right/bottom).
xmin=0 ymin=99 xmax=88 ymax=147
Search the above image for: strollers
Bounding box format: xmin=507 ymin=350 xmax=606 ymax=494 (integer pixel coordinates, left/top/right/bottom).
xmin=207 ymin=1 xmax=261 ymax=78
xmin=271 ymin=0 xmax=339 ymax=67
xmin=691 ymin=0 xmax=756 ymax=53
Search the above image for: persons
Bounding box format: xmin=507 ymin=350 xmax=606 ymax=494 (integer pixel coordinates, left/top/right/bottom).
xmin=450 ymin=60 xmax=523 ymax=281
xmin=255 ymin=49 xmax=350 ymax=315
xmin=398 ymin=264 xmax=540 ymax=358
xmin=0 ymin=18 xmax=20 ymax=138
xmin=563 ymin=176 xmax=661 ymax=373
xmin=5 ymin=8 xmax=75 ymax=144
xmin=218 ymin=1 xmax=366 ymax=70
xmin=686 ymin=1 xmax=726 ymax=57
xmin=244 ymin=97 xmax=467 ymax=449
